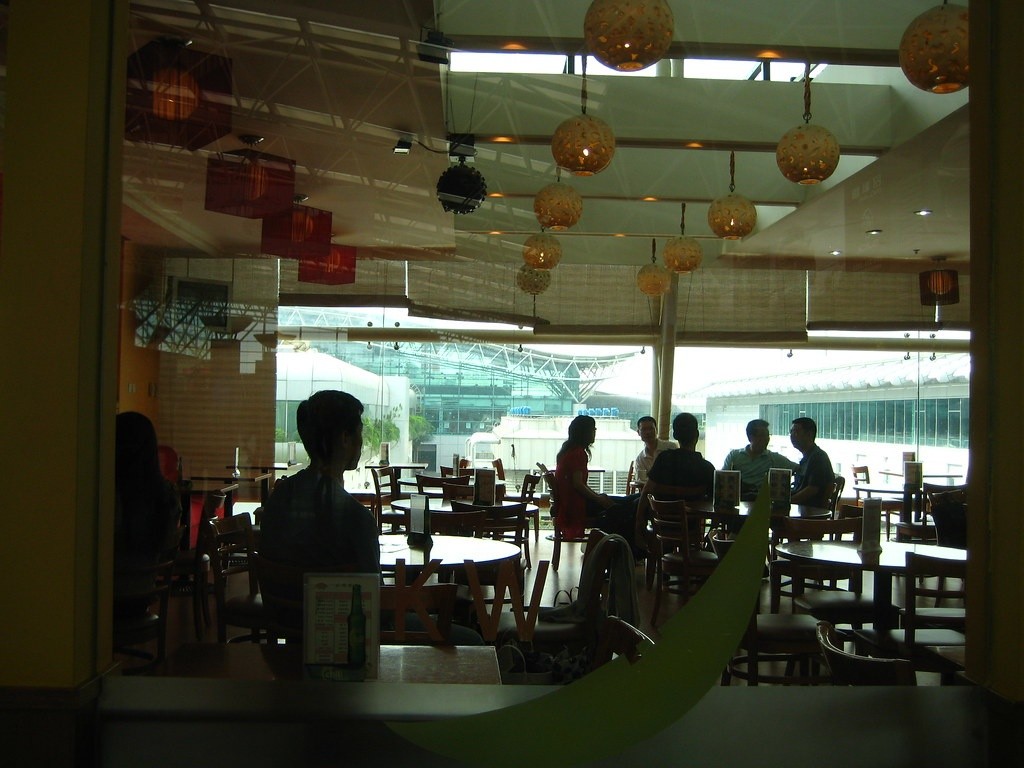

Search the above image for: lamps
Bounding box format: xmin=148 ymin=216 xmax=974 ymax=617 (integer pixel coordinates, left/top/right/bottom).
xmin=583 ymin=0 xmax=674 ymax=72
xmin=260 ymin=193 xmax=333 ymax=261
xmin=919 ymin=255 xmax=960 ymax=307
xmin=516 ymin=54 xmax=615 ymax=297
xmin=298 ymin=234 xmax=358 ymax=286
xmin=204 ymin=134 xmax=296 ymax=220
xmin=776 ymin=63 xmax=840 ymax=185
xmin=125 ymin=37 xmax=234 ymax=151
xmin=435 ymin=154 xmax=487 ymax=215
xmin=898 ymin=0 xmax=969 ymax=95
xmin=664 ymin=205 xmax=702 ymax=276
xmin=636 ymin=237 xmax=673 ymax=298
xmin=708 ymin=150 xmax=757 ymax=241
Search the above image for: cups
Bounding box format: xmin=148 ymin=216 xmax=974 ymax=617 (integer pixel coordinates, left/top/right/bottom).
xmin=637 ymin=470 xmax=646 ymax=482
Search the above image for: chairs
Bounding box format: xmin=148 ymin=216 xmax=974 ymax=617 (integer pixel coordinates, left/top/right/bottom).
xmin=116 ymin=460 xmax=970 ymax=688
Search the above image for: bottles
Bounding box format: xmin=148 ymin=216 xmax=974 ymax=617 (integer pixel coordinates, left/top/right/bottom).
xmin=347 ymin=584 xmax=366 ymax=666
xmin=914 ymin=466 xmax=921 ymax=485
xmin=423 ymin=496 xmax=431 ymax=534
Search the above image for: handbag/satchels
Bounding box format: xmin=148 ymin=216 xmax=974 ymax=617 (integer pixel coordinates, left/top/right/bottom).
xmin=497 ymin=637 xmax=555 ymax=685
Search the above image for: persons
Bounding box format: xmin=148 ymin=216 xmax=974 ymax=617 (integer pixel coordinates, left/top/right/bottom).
xmin=114 ymin=410 xmax=182 ymax=652
xmin=631 ymin=417 xmax=679 ymax=501
xmin=253 ymin=390 xmax=380 ymax=678
xmin=722 ymin=420 xmax=801 ymax=503
xmin=555 ymin=415 xmax=636 ymax=563
xmin=636 ymin=413 xmax=715 ymax=553
xmin=788 ymin=418 xmax=835 ymax=507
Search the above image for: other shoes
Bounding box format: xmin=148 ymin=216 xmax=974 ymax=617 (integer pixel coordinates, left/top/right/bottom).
xmin=763 ymin=564 xmax=770 ymax=577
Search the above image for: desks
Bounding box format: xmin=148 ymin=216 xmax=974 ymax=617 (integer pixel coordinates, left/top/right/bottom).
xmin=542 ymin=467 xmax=605 ymax=495
xmin=173 ymin=639 xmax=506 ymax=685
xmin=190 ymin=483 xmax=240 ymax=586
xmin=694 ymin=497 xmax=833 ymax=614
xmin=853 ymin=482 xmax=924 ymax=541
xmin=388 ymin=497 xmax=540 ymax=540
xmin=375 ymin=534 xmax=521 ymax=644
xmin=365 ymin=462 xmax=428 ymax=530
xmin=773 ymin=536 xmax=971 ymax=633
xmin=226 ymin=464 xmax=287 ymax=507
xmin=188 ymin=473 xmax=272 ymax=569
xmin=878 ymin=467 xmax=964 ymax=487
